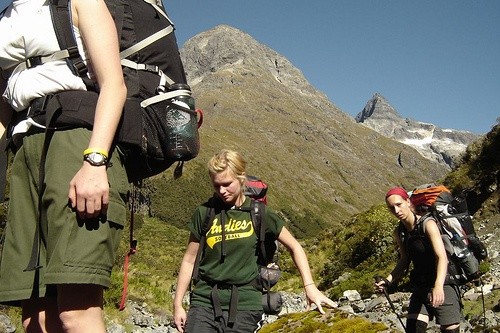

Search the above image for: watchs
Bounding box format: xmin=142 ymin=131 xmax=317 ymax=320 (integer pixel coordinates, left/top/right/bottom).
xmin=83 ymin=152 xmax=108 ymax=166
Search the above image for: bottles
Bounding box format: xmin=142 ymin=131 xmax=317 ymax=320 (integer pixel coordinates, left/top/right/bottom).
xmin=453 ymin=234 xmax=479 ymax=276
xmin=166 ymin=83 xmax=199 ymax=161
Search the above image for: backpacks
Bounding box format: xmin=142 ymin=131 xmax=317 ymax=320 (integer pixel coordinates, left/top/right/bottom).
xmin=399 ymin=184 xmax=487 ymax=286
xmin=200 ymin=175 xmax=280 ymax=292
xmin=0 ymin=0 xmax=200 ymax=183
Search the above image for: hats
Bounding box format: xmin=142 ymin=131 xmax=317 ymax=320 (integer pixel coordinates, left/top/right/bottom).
xmin=385 ymin=187 xmax=409 ymax=202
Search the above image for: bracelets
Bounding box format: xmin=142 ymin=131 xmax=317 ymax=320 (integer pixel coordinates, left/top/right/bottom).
xmin=83 ymin=148 xmax=109 ymax=158
xmin=383 ymin=278 xmax=391 ymax=287
xmin=304 ymin=282 xmax=315 ymax=288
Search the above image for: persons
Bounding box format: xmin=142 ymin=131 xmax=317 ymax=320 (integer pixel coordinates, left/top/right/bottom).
xmin=373 ymin=187 xmax=462 ymax=333
xmin=172 ymin=150 xmax=337 ymax=333
xmin=0 ymin=0 xmax=128 ymax=333
